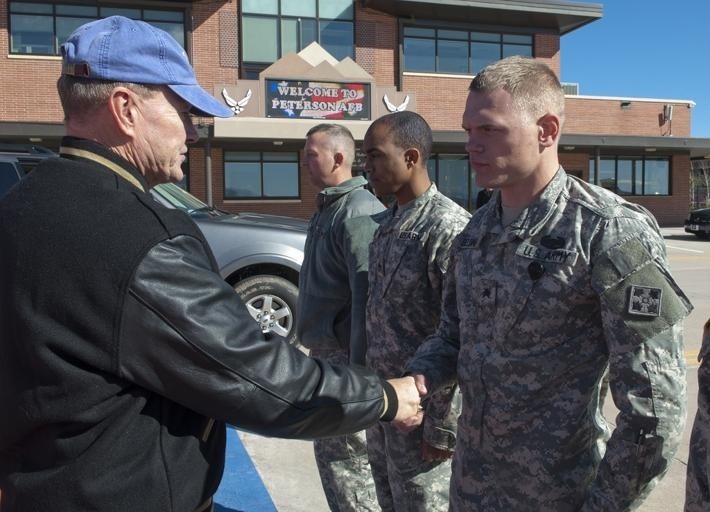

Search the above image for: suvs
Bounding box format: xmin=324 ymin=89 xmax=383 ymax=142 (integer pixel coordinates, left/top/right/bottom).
xmin=0 ymin=141 xmax=313 ymax=347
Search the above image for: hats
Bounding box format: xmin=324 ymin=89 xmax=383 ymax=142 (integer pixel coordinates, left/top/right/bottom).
xmin=60 ymin=15 xmax=235 ymax=118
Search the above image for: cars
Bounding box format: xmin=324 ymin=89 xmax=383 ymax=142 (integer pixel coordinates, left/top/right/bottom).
xmin=684 ymin=208 xmax=710 ymax=239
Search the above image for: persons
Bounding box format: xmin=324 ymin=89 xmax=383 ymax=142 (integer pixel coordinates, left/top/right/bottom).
xmin=294 ymin=123 xmax=389 ymax=512
xmin=684 ymin=318 xmax=710 ymax=512
xmin=388 ymin=57 xmax=695 ymax=512
xmin=476 ymin=188 xmax=494 ymax=208
xmin=0 ymin=14 xmax=422 ymax=512
xmin=364 ymin=110 xmax=474 ymax=512
